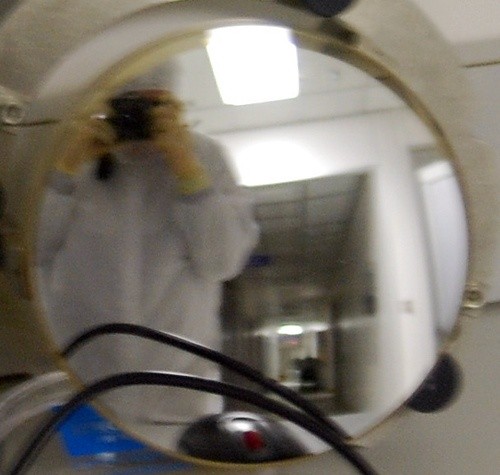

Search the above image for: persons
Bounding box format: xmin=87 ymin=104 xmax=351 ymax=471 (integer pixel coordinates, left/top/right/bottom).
xmin=35 ymin=55 xmax=261 ymax=453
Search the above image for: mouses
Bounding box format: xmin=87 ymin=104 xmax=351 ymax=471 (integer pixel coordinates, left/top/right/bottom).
xmin=178 ymin=408 xmax=312 ymax=462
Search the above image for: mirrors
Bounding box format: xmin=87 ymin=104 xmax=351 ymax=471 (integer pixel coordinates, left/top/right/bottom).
xmin=23 ymin=27 xmax=473 ymax=468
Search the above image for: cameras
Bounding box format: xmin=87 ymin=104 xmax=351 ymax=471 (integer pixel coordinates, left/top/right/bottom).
xmin=101 ymin=92 xmax=166 ymax=142
